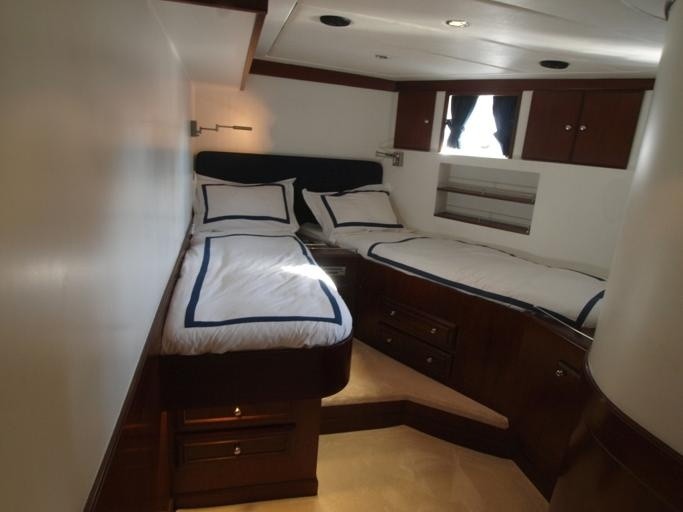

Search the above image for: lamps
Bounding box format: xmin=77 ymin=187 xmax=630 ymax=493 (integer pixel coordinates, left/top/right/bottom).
xmin=375 ymin=149 xmax=405 ymax=168
xmin=189 ymin=119 xmax=252 ymax=138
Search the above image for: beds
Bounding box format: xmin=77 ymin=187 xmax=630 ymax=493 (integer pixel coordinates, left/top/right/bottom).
xmin=303 ymin=223 xmax=607 ymax=502
xmin=162 ymin=230 xmax=353 ymax=507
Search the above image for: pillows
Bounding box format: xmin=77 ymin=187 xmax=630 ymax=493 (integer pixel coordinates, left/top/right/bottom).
xmin=189 ymin=171 xmax=300 ymax=235
xmin=303 ymin=182 xmax=411 ymax=243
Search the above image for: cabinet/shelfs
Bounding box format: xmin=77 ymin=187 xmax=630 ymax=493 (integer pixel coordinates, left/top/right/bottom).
xmin=433 ymin=162 xmax=541 ymax=235
